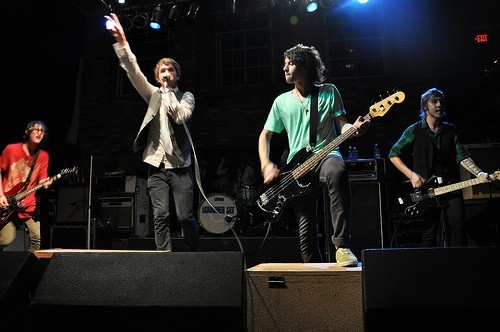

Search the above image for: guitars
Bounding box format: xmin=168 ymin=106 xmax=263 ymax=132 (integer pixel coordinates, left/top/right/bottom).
xmin=248 ymin=90 xmax=407 ymax=225
xmin=397 ymin=169 xmax=500 ymax=218
xmin=0 ymin=164 xmax=81 ymax=230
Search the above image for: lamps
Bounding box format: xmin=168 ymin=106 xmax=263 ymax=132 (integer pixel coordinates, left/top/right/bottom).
xmin=225 ymin=0 xmax=339 ymax=17
xmin=111 ymin=0 xmax=201 ymax=33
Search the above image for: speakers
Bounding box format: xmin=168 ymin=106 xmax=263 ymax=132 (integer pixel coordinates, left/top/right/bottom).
xmin=0 ymin=250 xmax=43 ymax=332
xmin=39 ymin=187 xmax=91 ymax=226
xmin=94 ymin=192 xmax=149 ymax=249
xmin=39 ymin=225 xmax=87 ymax=250
xmin=323 ymin=158 xmax=384 ymax=263
xmin=27 ymin=249 xmax=245 ymax=331
xmin=243 ymin=259 xmax=363 ymax=332
xmin=360 ymin=245 xmax=500 ymax=332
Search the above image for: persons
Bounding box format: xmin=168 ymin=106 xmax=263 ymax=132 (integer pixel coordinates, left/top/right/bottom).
xmin=388 ymin=87 xmax=489 ymax=248
xmin=104 ymin=11 xmax=201 ymax=251
xmin=217 ymin=157 xmax=254 ymax=180
xmin=258 ymin=43 xmax=372 ymax=267
xmin=0 ymin=120 xmax=52 ymax=251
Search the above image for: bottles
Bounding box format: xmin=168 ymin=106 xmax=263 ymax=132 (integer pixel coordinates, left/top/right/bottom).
xmin=373 ymin=143 xmax=380 ymax=159
xmin=348 ymin=147 xmax=358 ymax=161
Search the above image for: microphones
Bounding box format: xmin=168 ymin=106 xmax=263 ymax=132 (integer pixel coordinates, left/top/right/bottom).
xmin=162 ymin=75 xmax=170 ymax=82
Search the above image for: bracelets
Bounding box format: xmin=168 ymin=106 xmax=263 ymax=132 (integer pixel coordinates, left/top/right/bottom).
xmin=262 ymin=161 xmax=270 ymax=173
xmin=169 ymin=90 xmax=175 ymax=93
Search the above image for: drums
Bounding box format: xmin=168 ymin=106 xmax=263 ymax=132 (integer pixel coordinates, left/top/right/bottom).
xmin=198 ymin=192 xmax=239 ymax=236
xmin=236 ymin=185 xmax=257 ymax=202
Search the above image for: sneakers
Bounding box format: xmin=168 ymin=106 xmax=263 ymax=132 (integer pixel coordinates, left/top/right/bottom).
xmin=335 ymin=247 xmax=358 ymax=266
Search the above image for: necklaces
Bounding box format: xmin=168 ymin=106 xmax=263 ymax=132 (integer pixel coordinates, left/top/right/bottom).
xmin=297 ymin=93 xmax=310 ymax=116
